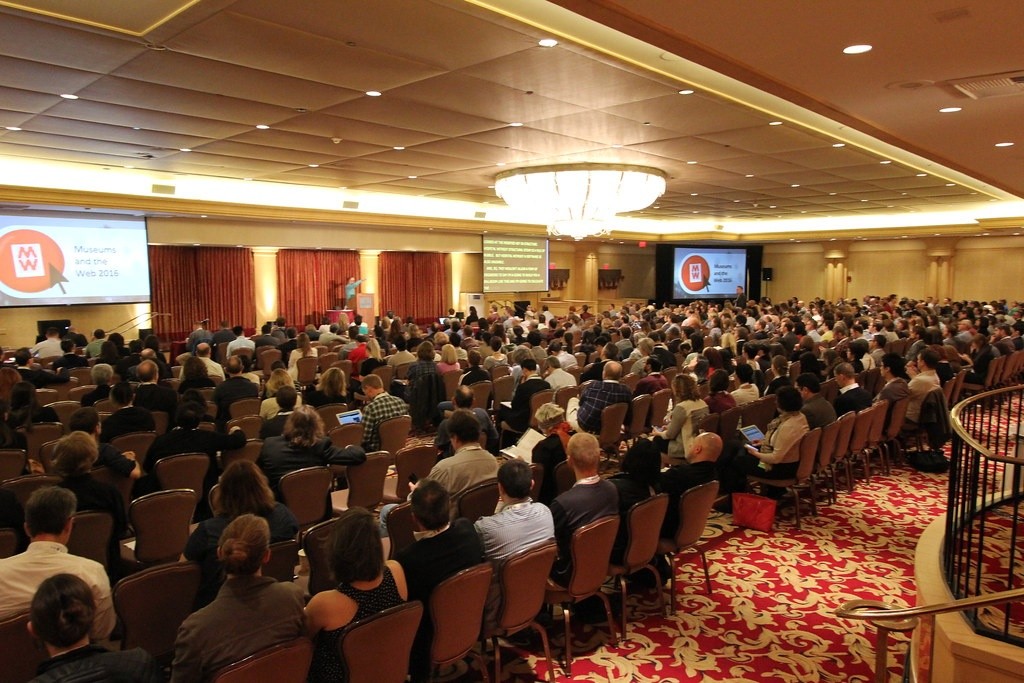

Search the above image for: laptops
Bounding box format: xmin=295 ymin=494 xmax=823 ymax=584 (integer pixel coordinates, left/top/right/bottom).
xmin=740 ymin=424 xmax=765 ymax=444
xmin=336 ymin=409 xmax=363 ymax=426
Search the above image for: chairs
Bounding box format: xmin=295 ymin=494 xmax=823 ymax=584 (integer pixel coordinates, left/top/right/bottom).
xmin=0 ymin=338 xmax=1024 ymax=683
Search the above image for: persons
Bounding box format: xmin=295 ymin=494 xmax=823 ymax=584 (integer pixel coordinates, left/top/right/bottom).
xmin=0 ymin=277 xmax=1024 ymax=683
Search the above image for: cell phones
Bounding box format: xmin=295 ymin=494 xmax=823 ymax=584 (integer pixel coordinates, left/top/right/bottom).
xmin=744 ymin=443 xmax=758 ymax=452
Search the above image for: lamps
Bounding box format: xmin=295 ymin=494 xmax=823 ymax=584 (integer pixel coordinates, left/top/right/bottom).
xmin=495 ymin=163 xmax=668 ymax=239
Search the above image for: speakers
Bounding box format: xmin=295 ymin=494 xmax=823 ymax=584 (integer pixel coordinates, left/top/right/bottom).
xmin=762 ymin=268 xmax=773 ymax=282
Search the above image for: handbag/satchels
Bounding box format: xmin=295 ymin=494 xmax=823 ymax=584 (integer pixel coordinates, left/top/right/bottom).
xmin=731 ymin=492 xmax=777 ymax=533
xmin=905 ymin=450 xmax=947 ymax=472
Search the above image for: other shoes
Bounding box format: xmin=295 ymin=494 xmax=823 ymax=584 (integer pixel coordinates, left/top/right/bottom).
xmin=715 ymin=495 xmax=732 ymax=514
